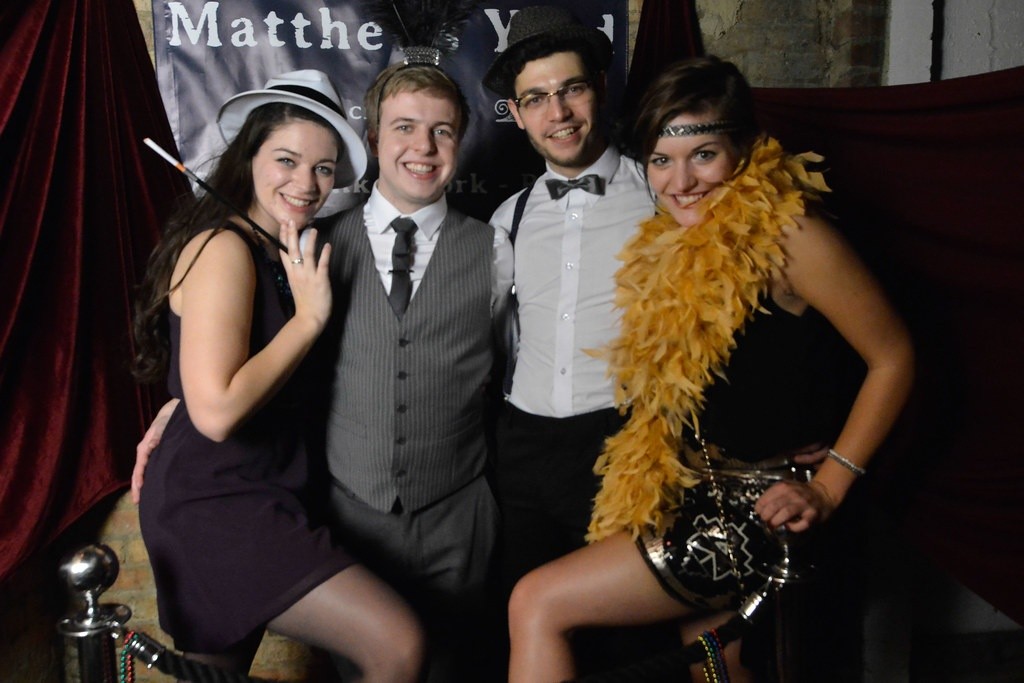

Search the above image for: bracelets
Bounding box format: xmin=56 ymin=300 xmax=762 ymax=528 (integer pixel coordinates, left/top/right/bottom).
xmin=827 ymin=449 xmax=866 ymax=476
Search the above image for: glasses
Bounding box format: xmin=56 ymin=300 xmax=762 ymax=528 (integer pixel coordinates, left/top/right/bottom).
xmin=512 ymin=79 xmax=595 ymax=112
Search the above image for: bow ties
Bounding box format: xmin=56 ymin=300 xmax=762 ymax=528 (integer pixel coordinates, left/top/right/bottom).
xmin=546 ymin=175 xmax=605 ymax=200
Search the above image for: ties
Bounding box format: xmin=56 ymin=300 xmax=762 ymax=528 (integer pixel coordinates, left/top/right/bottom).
xmin=388 ymin=217 xmax=417 ymax=326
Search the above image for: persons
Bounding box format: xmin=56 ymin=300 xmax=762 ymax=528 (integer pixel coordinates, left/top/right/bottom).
xmin=138 ymin=70 xmax=424 ymax=683
xmin=131 ymin=64 xmax=515 ymax=683
xmin=508 ymin=58 xmax=917 ymax=683
xmin=488 ymin=5 xmax=660 ymax=683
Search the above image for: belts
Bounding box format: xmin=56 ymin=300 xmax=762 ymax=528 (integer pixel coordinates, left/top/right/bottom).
xmin=751 ymin=444 xmax=830 ymax=470
xmin=500 ymin=405 xmax=628 ymax=439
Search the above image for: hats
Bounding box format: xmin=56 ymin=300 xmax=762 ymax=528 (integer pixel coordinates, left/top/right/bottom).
xmin=481 ymin=4 xmax=614 ymax=107
xmin=214 ymin=68 xmax=368 ymax=189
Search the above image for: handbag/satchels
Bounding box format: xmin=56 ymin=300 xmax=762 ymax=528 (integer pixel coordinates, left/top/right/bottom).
xmin=723 ymin=509 xmax=823 ymax=597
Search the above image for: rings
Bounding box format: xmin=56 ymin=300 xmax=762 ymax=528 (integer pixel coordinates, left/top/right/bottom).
xmin=291 ymin=259 xmax=304 ymax=264
